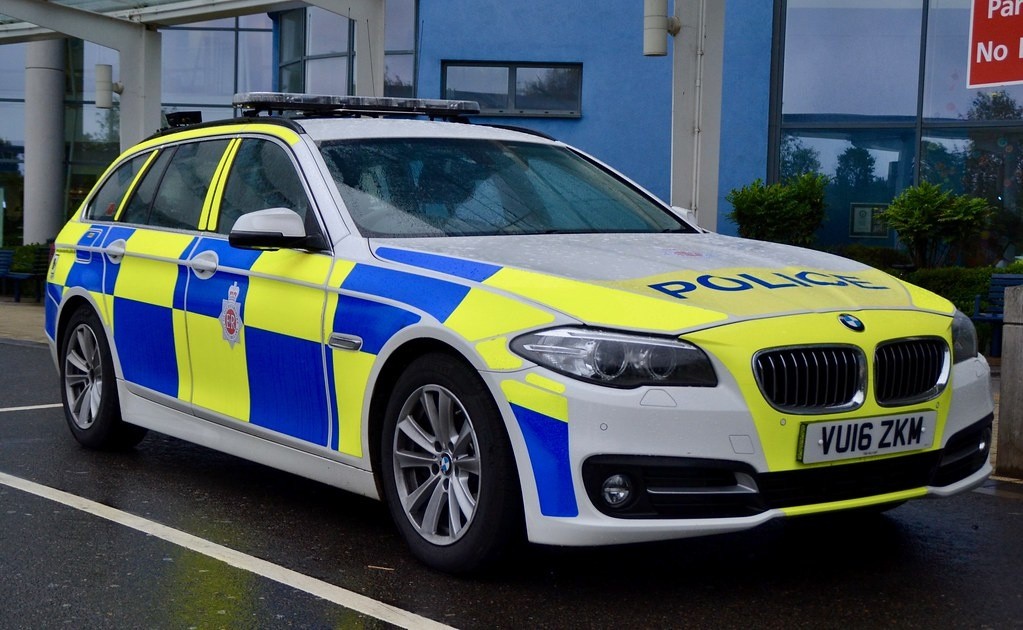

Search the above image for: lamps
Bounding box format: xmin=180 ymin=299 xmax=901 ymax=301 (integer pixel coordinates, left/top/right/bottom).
xmin=643 ymin=0 xmax=679 ymax=57
xmin=92 ymin=63 xmax=125 ymax=109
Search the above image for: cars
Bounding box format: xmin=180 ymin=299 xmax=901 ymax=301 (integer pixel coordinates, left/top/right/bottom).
xmin=41 ymin=83 xmax=997 ymax=581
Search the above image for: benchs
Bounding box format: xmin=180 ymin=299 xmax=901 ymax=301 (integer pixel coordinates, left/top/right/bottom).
xmin=0 ymin=244 xmax=52 ymax=303
